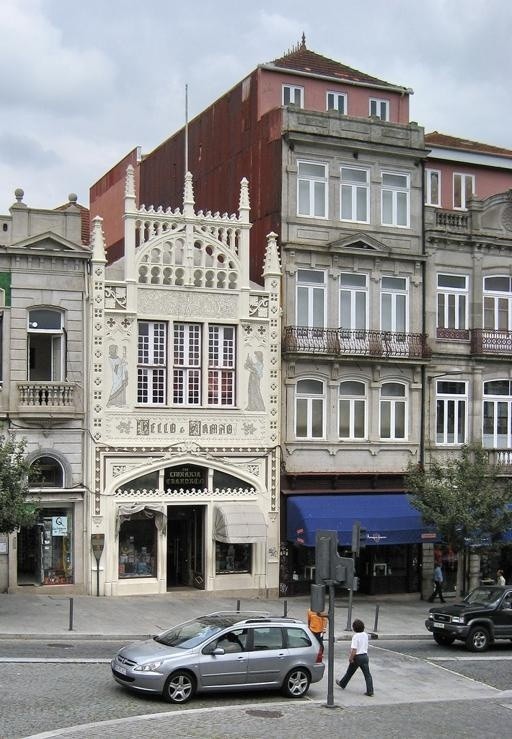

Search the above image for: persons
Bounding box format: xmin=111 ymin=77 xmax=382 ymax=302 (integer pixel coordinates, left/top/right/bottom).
xmin=335 ymin=619 xmax=375 ymax=697
xmin=244 ymin=351 xmax=267 ymax=411
xmin=427 ymin=560 xmax=446 ymax=603
xmin=107 ymin=345 xmax=131 ymax=408
xmin=307 ymin=609 xmax=328 ymax=653
xmin=494 ymin=568 xmax=506 ymax=586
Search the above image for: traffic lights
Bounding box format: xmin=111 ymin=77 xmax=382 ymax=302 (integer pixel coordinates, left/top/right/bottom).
xmin=353 ymin=577 xmax=360 ymax=591
xmin=352 ymin=521 xmax=368 ymax=557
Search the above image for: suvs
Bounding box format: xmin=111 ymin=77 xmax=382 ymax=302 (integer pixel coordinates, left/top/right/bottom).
xmin=426 ymin=584 xmax=512 ymax=652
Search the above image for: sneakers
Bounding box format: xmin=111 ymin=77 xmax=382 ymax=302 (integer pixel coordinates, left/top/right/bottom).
xmin=336 ymin=679 xmax=344 ymax=689
xmin=365 ymin=692 xmax=373 ymax=696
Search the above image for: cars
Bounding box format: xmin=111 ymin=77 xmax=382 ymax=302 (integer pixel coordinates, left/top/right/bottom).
xmin=109 ymin=610 xmax=326 ymax=705
